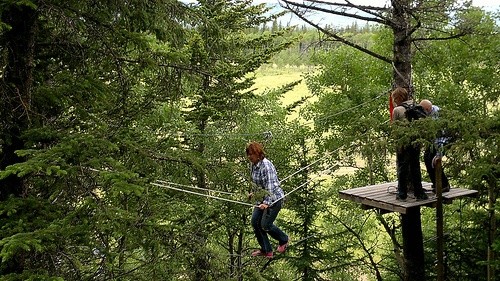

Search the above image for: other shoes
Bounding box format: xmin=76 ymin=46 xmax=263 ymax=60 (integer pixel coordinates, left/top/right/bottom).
xmin=432 ymin=185 xmax=450 ymax=193
xmin=277 ymin=238 xmax=290 ymax=252
xmin=252 ymin=250 xmax=273 ymax=258
xmin=395 ymin=194 xmax=407 ymax=202
xmin=416 ymin=195 xmax=428 ymax=201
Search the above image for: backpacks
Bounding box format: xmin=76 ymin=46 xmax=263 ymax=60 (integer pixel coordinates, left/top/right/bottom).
xmin=395 ymin=103 xmax=426 ymax=123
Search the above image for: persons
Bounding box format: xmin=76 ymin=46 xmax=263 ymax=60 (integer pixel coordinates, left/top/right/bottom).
xmin=391 ymin=87 xmax=428 ymax=201
xmin=245 ymin=141 xmax=290 ymax=255
xmin=419 ymin=99 xmax=450 ymax=193
